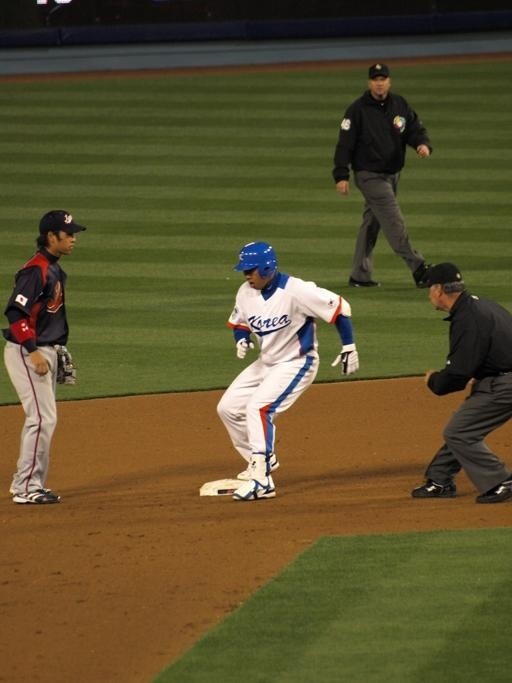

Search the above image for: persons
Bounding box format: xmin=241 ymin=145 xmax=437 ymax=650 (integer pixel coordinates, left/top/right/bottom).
xmin=332 ymin=64 xmax=437 ymax=288
xmin=2 ymin=210 xmax=88 ymax=505
xmin=411 ymin=261 xmax=512 ymax=503
xmin=215 ymin=242 xmax=359 ymax=501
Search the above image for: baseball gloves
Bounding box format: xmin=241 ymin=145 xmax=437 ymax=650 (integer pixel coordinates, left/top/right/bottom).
xmin=56 ymin=348 xmax=77 ymax=386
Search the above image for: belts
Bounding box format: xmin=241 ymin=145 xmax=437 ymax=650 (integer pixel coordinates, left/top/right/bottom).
xmin=6 ymin=335 xmax=54 ymax=347
xmin=473 ymin=368 xmax=512 ymax=381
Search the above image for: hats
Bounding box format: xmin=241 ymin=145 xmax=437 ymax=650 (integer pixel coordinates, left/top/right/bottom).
xmin=38 ymin=210 xmax=87 ymax=234
xmin=369 ymin=63 xmax=389 ymax=78
xmin=415 ymin=262 xmax=462 ymax=288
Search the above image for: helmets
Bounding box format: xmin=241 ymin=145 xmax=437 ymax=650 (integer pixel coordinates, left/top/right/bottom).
xmin=232 ymin=241 xmax=279 ymax=279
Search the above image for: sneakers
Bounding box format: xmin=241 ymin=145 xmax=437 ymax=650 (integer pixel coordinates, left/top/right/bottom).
xmin=348 ymin=275 xmax=381 ymax=287
xmin=476 ymin=474 xmax=512 ymax=504
xmin=8 ymin=488 xmax=61 ymax=504
xmin=235 ymin=452 xmax=280 ymax=480
xmin=411 ymin=478 xmax=456 ymax=498
xmin=413 ymin=261 xmax=434 ymax=289
xmin=231 ymin=474 xmax=277 ymax=501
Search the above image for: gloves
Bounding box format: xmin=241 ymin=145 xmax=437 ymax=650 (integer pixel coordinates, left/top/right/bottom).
xmin=235 ymin=337 xmax=255 ymax=359
xmin=330 ymin=342 xmax=359 ymax=376
